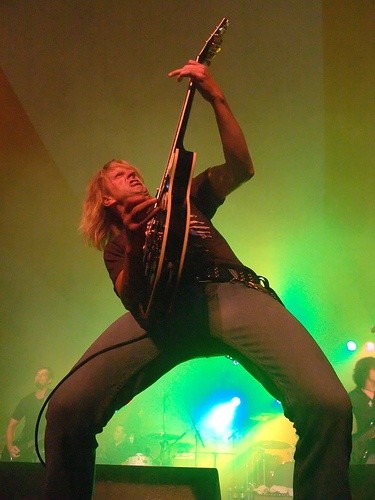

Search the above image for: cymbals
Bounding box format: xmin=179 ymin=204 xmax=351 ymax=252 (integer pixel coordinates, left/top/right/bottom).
xmin=148 ymin=432 xmax=181 ymax=442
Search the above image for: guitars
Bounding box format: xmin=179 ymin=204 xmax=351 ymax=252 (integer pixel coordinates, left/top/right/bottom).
xmin=1 ymin=431 xmax=45 ymax=462
xmin=348 ymin=425 xmax=375 ymax=465
xmin=136 ymin=17 xmax=231 ymax=323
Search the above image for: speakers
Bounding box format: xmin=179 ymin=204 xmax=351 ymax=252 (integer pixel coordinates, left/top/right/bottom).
xmin=91 ymin=464 xmax=223 ymax=500
xmin=0 ymin=461 xmax=47 ymax=500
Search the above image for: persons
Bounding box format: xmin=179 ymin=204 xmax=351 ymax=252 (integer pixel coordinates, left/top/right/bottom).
xmin=348 ymin=357 xmax=375 ymax=465
xmin=7 ymin=367 xmax=54 ymax=461
xmin=43 ymin=60 xmax=355 ymax=500
